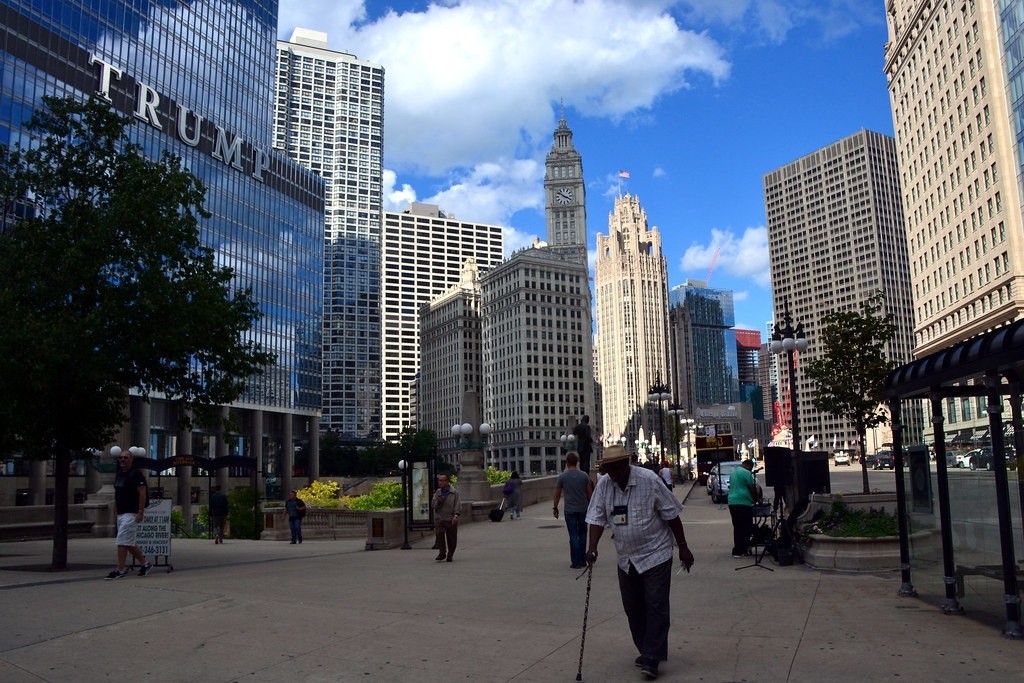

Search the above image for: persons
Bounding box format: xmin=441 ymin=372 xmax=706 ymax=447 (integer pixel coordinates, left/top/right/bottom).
xmin=282 ymin=491 xmax=306 ymax=544
xmin=508 ymin=471 xmax=523 ymax=520
xmin=585 ymin=445 xmax=695 ymax=677
xmin=728 ymin=460 xmax=764 ymax=559
xmin=106 ymin=450 xmax=152 ymax=580
xmin=431 ymin=473 xmax=461 ymax=562
xmin=553 ymin=453 xmax=592 ymax=569
xmin=209 ymin=485 xmax=228 ymax=544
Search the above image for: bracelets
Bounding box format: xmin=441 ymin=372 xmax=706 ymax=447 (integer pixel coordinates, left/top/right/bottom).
xmin=553 ymin=507 xmax=557 ymax=510
xmin=678 ymin=540 xmax=687 ymax=546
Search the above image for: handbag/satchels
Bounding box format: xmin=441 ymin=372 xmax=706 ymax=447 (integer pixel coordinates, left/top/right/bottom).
xmin=297 ymin=499 xmax=307 ymax=518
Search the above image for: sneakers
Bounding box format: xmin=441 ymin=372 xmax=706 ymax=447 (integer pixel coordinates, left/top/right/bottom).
xmin=137 ymin=562 xmax=153 ymax=577
xmin=105 ymin=570 xmax=125 ymax=580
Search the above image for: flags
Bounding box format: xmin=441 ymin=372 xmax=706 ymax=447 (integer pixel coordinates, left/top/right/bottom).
xmin=619 ymin=170 xmax=630 ymax=179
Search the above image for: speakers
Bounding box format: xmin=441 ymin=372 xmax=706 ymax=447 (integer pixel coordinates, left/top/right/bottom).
xmin=763 ymin=446 xmax=793 ymax=486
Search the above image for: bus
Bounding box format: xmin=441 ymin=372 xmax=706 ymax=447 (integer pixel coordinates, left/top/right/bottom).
xmin=694 ymin=422 xmax=736 ymax=486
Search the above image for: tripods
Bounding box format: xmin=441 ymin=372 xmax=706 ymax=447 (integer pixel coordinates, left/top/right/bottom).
xmin=734 ymin=473 xmax=804 ymax=572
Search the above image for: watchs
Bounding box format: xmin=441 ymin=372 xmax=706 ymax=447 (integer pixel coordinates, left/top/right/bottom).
xmin=454 ymin=514 xmax=459 ymax=518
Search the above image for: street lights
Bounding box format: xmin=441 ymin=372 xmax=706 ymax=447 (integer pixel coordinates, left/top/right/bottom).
xmin=668 ymin=398 xmax=684 ymax=485
xmin=635 ymin=428 xmax=649 ymax=463
xmin=560 ymin=416 xmax=580 ymax=471
xmin=648 ymin=434 xmax=660 ymax=460
xmin=770 ymin=300 xmax=807 ymax=503
xmin=450 ymin=390 xmax=491 ymax=501
xmin=648 ymin=370 xmax=672 ymax=466
xmin=398 ymin=460 xmax=412 ymax=550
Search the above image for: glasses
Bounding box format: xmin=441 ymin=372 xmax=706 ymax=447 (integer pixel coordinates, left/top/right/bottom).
xmin=119 ymin=456 xmax=131 ymax=459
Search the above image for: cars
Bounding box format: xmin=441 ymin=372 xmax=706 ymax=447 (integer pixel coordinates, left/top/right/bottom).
xmin=956 ymin=449 xmax=983 ymax=468
xmin=865 ymin=454 xmax=895 ymax=470
xmin=835 ymin=454 xmax=850 ymax=466
xmin=883 ymin=449 xmax=910 ymax=467
xmin=969 ymin=446 xmax=1017 ymax=471
xmin=706 ymin=462 xmax=763 ymax=504
xmin=946 ymin=451 xmax=959 ymax=464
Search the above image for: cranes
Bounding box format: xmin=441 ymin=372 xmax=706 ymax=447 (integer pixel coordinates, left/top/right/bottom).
xmin=706 ymin=248 xmax=721 ymax=283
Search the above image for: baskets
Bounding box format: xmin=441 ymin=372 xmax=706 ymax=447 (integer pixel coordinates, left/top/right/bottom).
xmin=752 ymin=503 xmax=771 ymax=518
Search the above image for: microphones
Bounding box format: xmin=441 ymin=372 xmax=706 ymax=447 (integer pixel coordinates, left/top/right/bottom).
xmin=751 ymin=467 xmax=764 ymax=474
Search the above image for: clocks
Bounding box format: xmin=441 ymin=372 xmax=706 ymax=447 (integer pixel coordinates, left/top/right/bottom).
xmin=555 ymin=188 xmax=573 ymax=206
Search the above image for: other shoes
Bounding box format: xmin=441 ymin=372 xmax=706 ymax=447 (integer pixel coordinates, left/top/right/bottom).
xmin=733 ymin=552 xmax=745 ymax=557
xmin=517 ymin=517 xmax=521 ymax=520
xmin=220 ymin=539 xmax=222 ymax=544
xmin=215 ymin=535 xmax=218 ymax=544
xmin=640 ymin=657 xmax=659 ymax=678
xmin=290 ymin=541 xmax=296 ymax=544
xmin=570 ymin=562 xmax=587 ymax=569
xmin=300 ymin=537 xmax=303 ymax=544
xmin=634 ymin=654 xmax=645 ymax=667
xmin=510 ymin=513 xmax=513 ymax=520
xmin=436 ymin=552 xmax=446 ymax=560
xmin=446 ymin=557 xmax=452 ymax=562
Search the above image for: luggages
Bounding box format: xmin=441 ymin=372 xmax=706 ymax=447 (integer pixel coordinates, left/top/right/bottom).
xmin=488 ymin=496 xmax=508 ymax=522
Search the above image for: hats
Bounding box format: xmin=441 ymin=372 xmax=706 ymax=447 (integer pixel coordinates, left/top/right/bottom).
xmin=595 ymin=444 xmax=632 ymax=464
